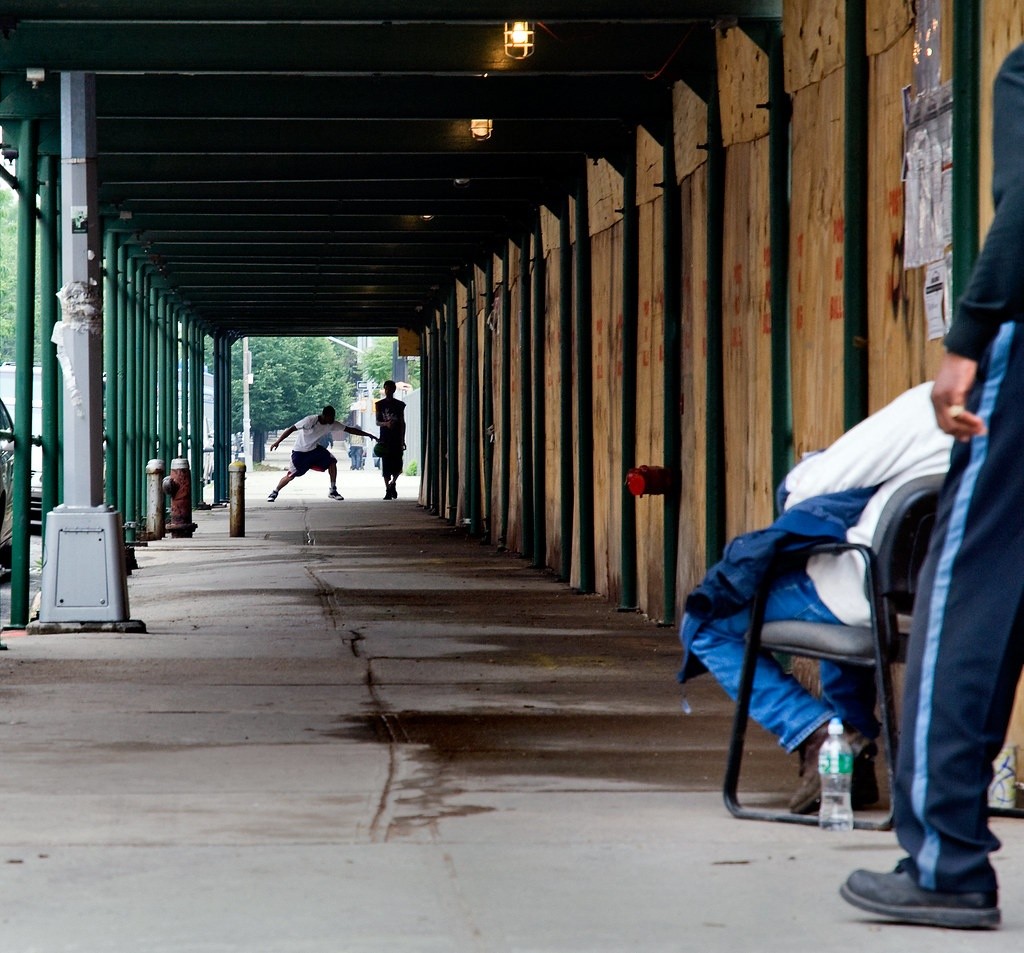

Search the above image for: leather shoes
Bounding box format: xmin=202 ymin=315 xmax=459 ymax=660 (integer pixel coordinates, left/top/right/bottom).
xmin=840 ymin=862 xmax=1001 ymax=928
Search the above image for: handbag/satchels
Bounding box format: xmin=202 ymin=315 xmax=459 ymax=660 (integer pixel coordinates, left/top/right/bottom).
xmin=374 ymin=438 xmax=388 ymax=458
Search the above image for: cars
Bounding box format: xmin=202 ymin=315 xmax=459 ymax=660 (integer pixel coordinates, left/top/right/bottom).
xmin=0 ymin=361 xmax=106 ymax=569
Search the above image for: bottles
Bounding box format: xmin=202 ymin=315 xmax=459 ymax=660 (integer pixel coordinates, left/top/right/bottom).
xmin=818 ymin=718 xmax=852 ymax=832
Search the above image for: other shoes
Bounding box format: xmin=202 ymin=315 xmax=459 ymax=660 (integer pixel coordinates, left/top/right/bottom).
xmin=383 ymin=481 xmax=397 ymax=500
xmin=788 ymin=716 xmax=874 ymax=815
xmin=852 ymin=757 xmax=881 ymax=810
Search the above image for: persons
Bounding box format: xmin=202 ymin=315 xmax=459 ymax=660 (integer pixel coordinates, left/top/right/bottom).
xmin=374 ymin=380 xmax=406 ymax=500
xmin=317 ymin=432 xmax=333 ymax=449
xmin=350 ymin=425 xmax=364 ymax=471
xmin=267 ymin=405 xmax=378 ymax=502
xmin=675 ymin=39 xmax=1024 ymax=928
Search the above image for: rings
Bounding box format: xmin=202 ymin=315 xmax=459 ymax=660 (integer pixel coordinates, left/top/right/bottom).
xmin=947 ymin=405 xmax=965 ymax=418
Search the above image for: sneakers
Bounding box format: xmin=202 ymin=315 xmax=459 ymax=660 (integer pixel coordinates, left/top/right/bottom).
xmin=267 ymin=491 xmax=279 ymax=502
xmin=328 ymin=490 xmax=344 ymax=501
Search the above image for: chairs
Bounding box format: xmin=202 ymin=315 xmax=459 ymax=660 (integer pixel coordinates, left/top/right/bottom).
xmin=723 ymin=471 xmax=1024 ymax=829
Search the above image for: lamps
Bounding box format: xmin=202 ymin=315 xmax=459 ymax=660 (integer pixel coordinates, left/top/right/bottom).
xmin=503 ymin=21 xmax=536 ymax=58
xmin=469 ymin=118 xmax=493 ymax=140
